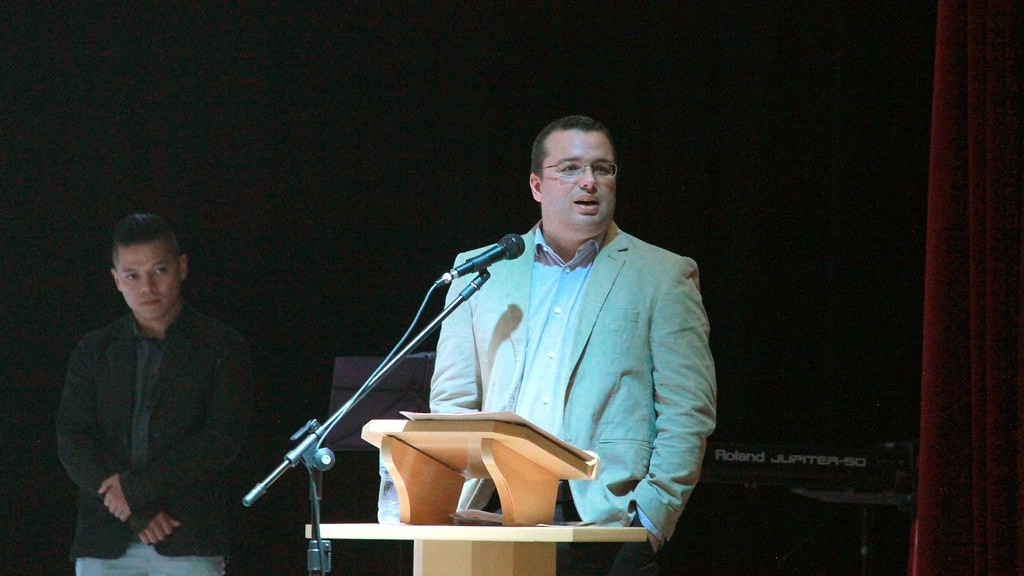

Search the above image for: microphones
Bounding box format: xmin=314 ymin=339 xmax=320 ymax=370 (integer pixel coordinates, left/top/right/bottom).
xmin=434 ymin=233 xmax=525 ymax=289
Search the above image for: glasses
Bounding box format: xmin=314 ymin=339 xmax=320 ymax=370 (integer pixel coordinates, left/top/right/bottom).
xmin=533 ymin=160 xmax=617 ymax=179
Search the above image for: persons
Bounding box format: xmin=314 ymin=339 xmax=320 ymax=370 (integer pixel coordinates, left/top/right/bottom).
xmin=56 ymin=215 xmax=254 ymax=576
xmin=427 ymin=114 xmax=717 ymax=576
